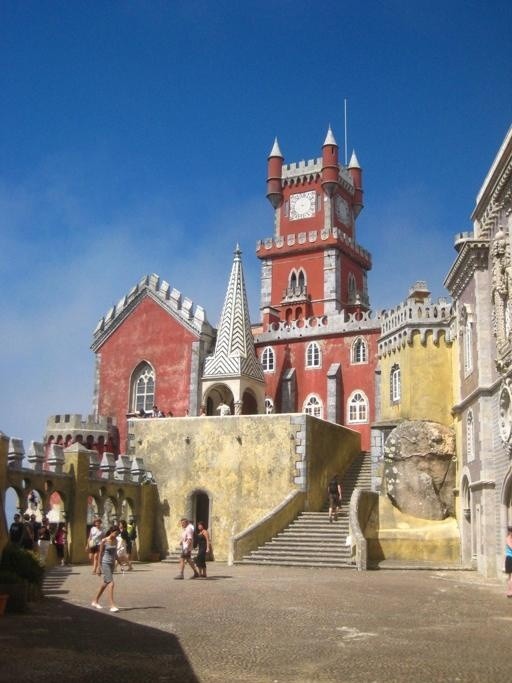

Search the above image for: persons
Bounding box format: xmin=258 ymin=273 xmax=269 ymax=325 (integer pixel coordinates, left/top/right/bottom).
xmin=10 ymin=513 xmax=137 ymax=612
xmin=328 ymin=474 xmax=343 ymax=522
xmin=503 ymin=525 xmax=512 ymax=597
xmin=196 ymin=521 xmax=210 ymax=577
xmin=173 ymin=519 xmax=199 ymax=579
xmin=135 ymin=400 xmax=231 ymax=418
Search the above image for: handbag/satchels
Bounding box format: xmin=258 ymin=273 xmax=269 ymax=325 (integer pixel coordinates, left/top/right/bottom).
xmin=87 ymin=540 xmax=96 ymax=548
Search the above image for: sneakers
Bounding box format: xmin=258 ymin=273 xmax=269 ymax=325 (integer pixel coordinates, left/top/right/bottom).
xmin=190 ymin=573 xmax=198 ymax=579
xmin=174 ymin=575 xmax=184 ymax=579
xmin=109 ymin=607 xmax=119 ymax=611
xmin=92 ymin=602 xmax=102 ymax=609
